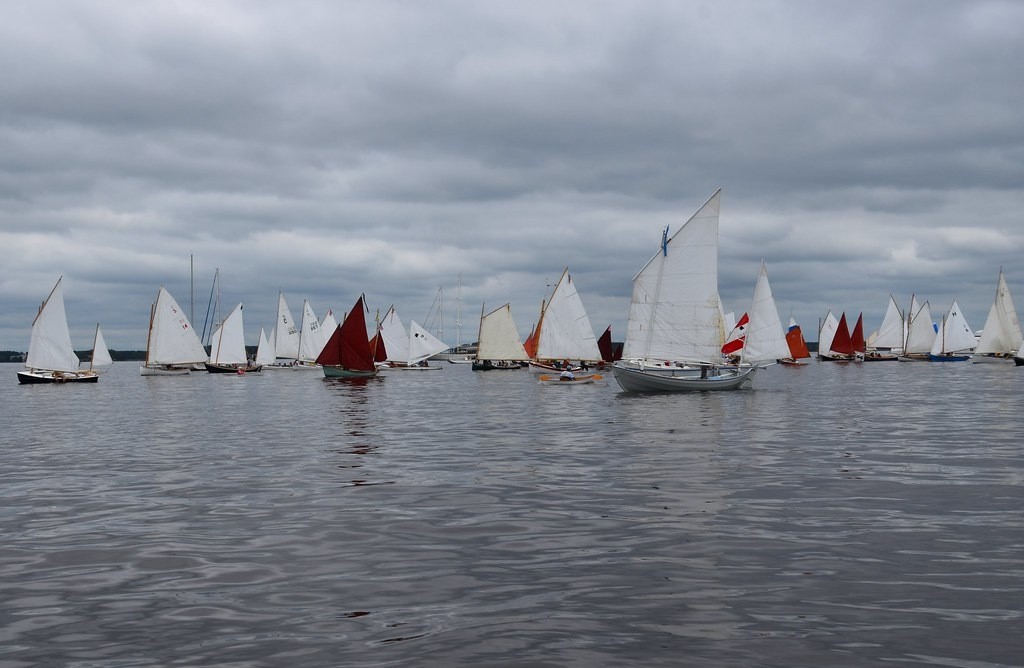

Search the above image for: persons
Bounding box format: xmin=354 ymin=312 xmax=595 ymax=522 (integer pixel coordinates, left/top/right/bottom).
xmin=483 ymin=358 xmax=518 ymax=367
xmin=246 ymin=357 xmax=251 ymax=368
xmin=418 ymin=360 xmax=428 ymax=367
xmin=559 ymin=365 xmax=575 ymax=382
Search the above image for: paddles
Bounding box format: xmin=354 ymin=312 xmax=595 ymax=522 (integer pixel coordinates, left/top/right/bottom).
xmin=539 ymin=375 xmax=604 ymax=381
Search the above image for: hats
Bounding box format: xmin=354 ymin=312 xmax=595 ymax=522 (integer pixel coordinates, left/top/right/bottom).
xmin=567 ymin=365 xmax=571 ymax=370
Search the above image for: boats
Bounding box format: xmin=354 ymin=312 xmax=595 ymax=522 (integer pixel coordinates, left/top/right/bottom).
xmin=448 ymin=358 xmax=479 ymax=366
xmin=537 ymin=372 xmax=601 ymax=384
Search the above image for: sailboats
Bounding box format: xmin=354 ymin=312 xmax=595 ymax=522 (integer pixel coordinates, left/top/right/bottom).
xmin=471 ymin=300 xmax=532 ymax=370
xmin=608 ymin=185 xmax=762 ymax=391
xmin=523 ymin=266 xmax=606 ymax=374
xmin=316 ymin=291 xmax=384 ymax=378
xmin=15 ymin=275 xmax=116 ymax=386
xmin=138 ymin=286 xmax=206 ymax=376
xmin=722 ymin=260 xmax=1024 ymax=367
xmin=197 ymin=265 xmax=452 ymax=370
